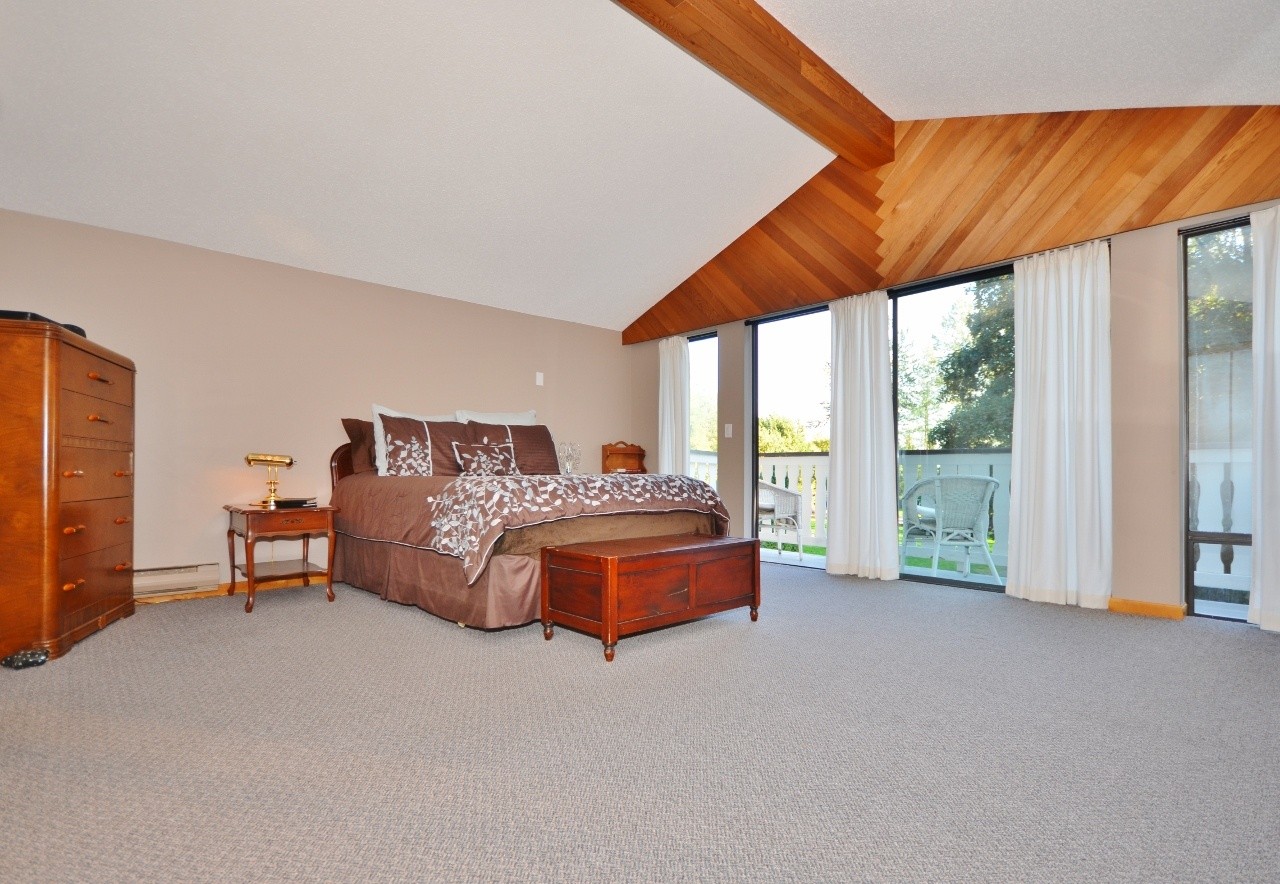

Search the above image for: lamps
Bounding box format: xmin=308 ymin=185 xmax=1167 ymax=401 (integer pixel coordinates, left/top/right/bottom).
xmin=246 ymin=454 xmax=294 ymax=506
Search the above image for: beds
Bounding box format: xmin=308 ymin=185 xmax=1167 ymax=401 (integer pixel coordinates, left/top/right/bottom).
xmin=328 ymin=443 xmax=731 ymax=631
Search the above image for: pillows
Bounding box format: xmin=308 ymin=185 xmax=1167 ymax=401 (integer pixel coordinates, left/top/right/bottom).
xmin=378 ymin=414 xmax=467 ymax=477
xmin=468 ymin=419 xmax=561 ymax=475
xmin=451 ymin=439 xmax=522 ymax=476
xmin=340 ymin=418 xmax=377 ymax=474
xmin=372 ymin=404 xmax=456 ymax=477
xmin=456 ymin=409 xmax=536 ymax=426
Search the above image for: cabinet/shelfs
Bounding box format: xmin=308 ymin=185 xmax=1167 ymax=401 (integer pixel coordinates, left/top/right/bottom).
xmin=0 ymin=318 xmax=136 ymax=660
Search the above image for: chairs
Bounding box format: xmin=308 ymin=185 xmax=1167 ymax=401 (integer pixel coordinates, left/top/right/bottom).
xmin=758 ymin=477 xmax=804 ymax=562
xmin=899 ymin=473 xmax=1004 ymax=586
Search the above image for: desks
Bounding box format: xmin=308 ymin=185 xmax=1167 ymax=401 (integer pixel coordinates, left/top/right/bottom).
xmin=540 ymin=532 xmax=762 ymax=662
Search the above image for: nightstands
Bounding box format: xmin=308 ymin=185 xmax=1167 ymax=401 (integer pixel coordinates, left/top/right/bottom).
xmin=221 ymin=502 xmax=341 ymax=613
